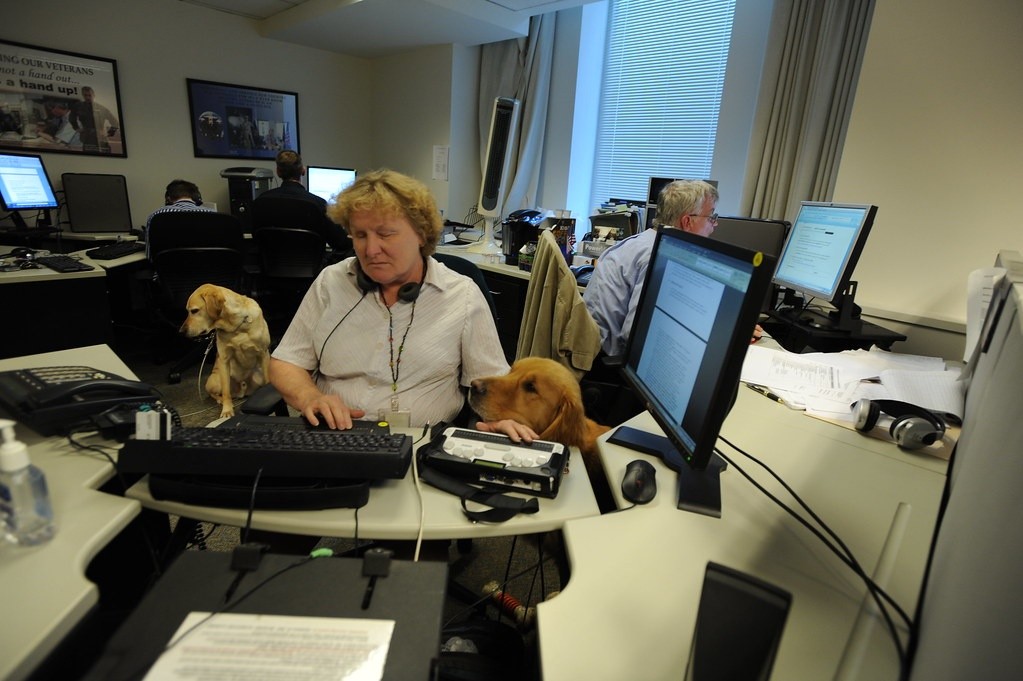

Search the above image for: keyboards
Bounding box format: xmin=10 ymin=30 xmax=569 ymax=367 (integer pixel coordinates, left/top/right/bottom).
xmin=87 ymin=241 xmax=146 ymax=260
xmin=38 ymin=255 xmax=95 ymax=273
xmin=124 ymin=414 xmax=415 ymax=480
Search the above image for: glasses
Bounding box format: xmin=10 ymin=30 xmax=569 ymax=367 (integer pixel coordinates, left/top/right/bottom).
xmin=689 ymin=214 xmax=718 ymax=226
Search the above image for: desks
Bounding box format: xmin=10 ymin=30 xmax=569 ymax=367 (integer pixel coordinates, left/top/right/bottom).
xmin=0 ymin=343 xmax=1023 ymax=681
xmin=0 ymin=224 xmax=599 ymax=295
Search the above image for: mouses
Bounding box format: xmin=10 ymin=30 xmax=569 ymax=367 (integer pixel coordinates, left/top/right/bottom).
xmin=621 ymin=459 xmax=657 ymax=505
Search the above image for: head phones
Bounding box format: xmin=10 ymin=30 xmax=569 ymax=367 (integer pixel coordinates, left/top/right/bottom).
xmin=164 ymin=192 xmax=202 ymax=205
xmin=357 ymin=256 xmax=427 ymax=301
xmin=852 ymin=399 xmax=946 ymax=450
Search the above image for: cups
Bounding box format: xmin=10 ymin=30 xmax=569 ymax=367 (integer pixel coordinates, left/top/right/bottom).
xmin=556 ymin=209 xmax=571 ymax=220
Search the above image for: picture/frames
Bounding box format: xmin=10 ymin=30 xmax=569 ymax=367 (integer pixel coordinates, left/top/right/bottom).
xmin=0 ymin=39 xmax=129 ymax=159
xmin=185 ymin=78 xmax=302 ymax=162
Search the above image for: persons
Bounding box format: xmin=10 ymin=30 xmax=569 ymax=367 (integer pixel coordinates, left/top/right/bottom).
xmin=240 ymin=150 xmax=354 ymax=254
xmin=583 ymin=179 xmax=763 ymax=390
xmin=239 ymin=168 xmax=540 ymax=564
xmin=145 ymin=179 xmax=219 ymax=283
xmin=34 ymin=85 xmax=119 ymax=150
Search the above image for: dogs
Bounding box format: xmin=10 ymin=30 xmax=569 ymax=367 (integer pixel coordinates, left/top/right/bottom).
xmin=179 ymin=284 xmax=271 ymax=419
xmin=468 ymin=355 xmax=611 ymax=457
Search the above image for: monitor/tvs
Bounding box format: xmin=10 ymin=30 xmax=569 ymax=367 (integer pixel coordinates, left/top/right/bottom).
xmin=0 ymin=152 xmax=60 ymax=212
xmin=772 ymin=200 xmax=879 ymax=333
xmin=606 ymin=224 xmax=777 ymax=518
xmin=306 ymin=165 xmax=358 ymax=205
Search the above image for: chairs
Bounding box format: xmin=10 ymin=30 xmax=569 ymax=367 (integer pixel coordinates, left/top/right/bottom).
xmin=511 ymin=231 xmax=639 ymax=423
xmin=149 ymin=211 xmax=241 ymax=333
xmin=244 ymin=254 xmax=494 ymax=426
xmin=251 ymin=195 xmax=327 ymax=332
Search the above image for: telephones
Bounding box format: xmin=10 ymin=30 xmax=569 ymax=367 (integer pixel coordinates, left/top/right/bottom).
xmin=0 ymin=366 xmax=160 ymax=437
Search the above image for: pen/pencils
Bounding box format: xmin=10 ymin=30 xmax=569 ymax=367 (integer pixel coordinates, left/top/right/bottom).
xmin=747 ymin=383 xmax=784 ymax=403
